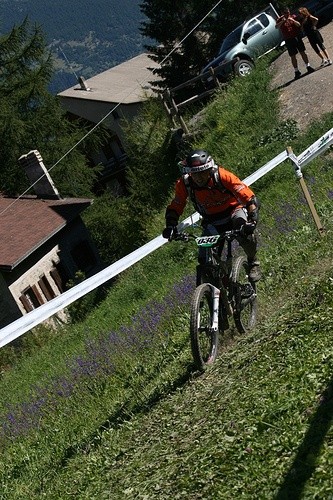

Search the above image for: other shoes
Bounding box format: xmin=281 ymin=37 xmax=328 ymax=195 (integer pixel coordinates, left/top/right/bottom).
xmin=295 ymin=71 xmax=301 ymax=80
xmin=307 ymin=66 xmax=315 ymax=74
xmin=327 ymin=57 xmax=332 ymax=64
xmin=320 ymin=59 xmax=327 ymax=66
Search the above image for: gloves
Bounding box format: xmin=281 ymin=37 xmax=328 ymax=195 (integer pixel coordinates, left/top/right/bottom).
xmin=240 ymin=221 xmax=255 ymax=236
xmin=163 ymin=227 xmax=178 ymax=242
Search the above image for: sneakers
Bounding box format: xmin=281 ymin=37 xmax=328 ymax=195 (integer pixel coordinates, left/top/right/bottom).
xmin=248 ymin=261 xmax=262 ymax=282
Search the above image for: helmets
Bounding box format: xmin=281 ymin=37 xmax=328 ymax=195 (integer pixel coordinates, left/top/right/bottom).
xmin=177 ymin=150 xmax=214 ymax=173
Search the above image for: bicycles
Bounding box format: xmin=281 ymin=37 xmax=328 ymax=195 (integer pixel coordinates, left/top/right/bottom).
xmin=168 ymin=224 xmax=260 ymax=372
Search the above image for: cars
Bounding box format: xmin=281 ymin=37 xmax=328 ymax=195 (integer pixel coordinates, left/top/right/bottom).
xmin=203 ymin=11 xmax=288 ymax=78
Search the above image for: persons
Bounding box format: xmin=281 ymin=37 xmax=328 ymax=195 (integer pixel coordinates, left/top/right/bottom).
xmin=163 ymin=151 xmax=261 ymax=328
xmin=275 ymin=7 xmax=315 ymax=79
xmin=298 ymin=8 xmax=332 ymax=67
